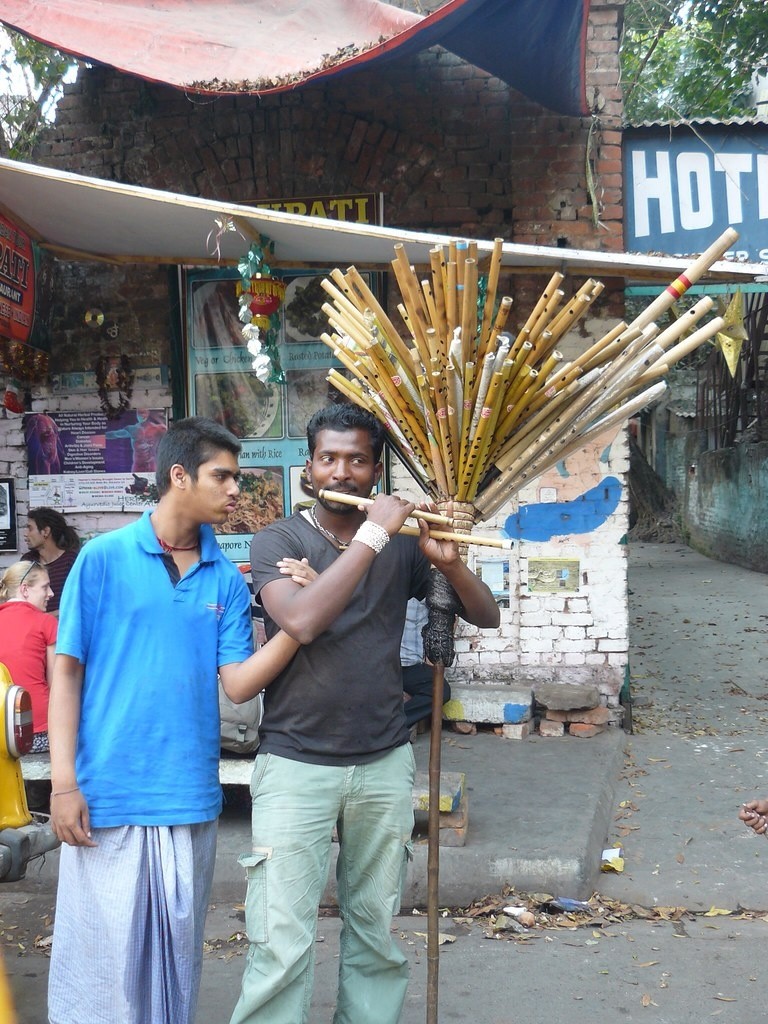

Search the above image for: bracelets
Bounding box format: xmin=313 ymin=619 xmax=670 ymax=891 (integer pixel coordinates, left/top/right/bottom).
xmin=352 ymin=520 xmax=390 ymax=554
xmin=51 ymin=787 xmax=80 ymax=796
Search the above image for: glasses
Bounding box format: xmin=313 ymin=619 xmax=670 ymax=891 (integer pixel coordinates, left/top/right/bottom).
xmin=20 ymin=560 xmax=41 ymax=584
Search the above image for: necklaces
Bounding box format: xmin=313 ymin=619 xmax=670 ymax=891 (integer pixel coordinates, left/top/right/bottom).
xmin=311 ymin=502 xmax=370 ymax=550
xmin=34 ymin=549 xmax=65 ymax=566
xmin=156 ymin=534 xmax=198 ymax=553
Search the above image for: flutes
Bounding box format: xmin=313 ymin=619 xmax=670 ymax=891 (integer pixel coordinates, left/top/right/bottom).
xmin=319 ymin=486 xmax=455 ymax=526
xmin=396 ymin=526 xmax=516 ymax=550
xmin=317 ymin=226 xmax=740 ymax=526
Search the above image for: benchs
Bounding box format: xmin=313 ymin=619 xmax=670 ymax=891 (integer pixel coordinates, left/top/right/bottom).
xmin=19 ymin=748 xmax=470 ymax=846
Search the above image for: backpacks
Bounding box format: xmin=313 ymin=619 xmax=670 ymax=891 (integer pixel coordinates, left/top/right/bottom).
xmin=219 ymin=674 xmax=263 ymax=757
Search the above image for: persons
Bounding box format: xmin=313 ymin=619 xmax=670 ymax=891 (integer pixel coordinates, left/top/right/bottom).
xmin=0 ymin=507 xmax=85 ymax=752
xmin=738 ymin=799 xmax=768 ymax=836
xmin=47 ymin=417 xmax=323 ymax=1024
xmin=228 ymin=403 xmax=501 ymax=1024
xmin=391 ymin=524 xmax=460 ymax=731
